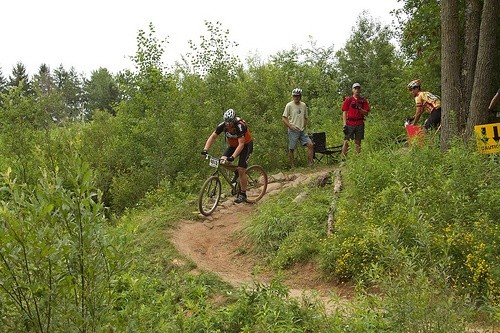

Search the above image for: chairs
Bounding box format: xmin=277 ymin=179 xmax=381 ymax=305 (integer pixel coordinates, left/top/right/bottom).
xmin=312 ymin=131 xmax=344 ymax=165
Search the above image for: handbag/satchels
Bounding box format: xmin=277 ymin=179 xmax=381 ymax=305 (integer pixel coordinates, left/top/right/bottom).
xmin=406 ymin=125 xmax=423 ymax=147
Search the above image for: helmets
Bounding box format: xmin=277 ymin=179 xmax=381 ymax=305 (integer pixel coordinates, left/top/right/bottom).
xmin=224 ymin=108 xmax=236 ymax=121
xmin=408 ymin=79 xmax=421 ymax=87
xmin=291 ymin=88 xmax=303 ymax=96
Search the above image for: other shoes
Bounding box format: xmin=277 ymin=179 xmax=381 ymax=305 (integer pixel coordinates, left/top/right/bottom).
xmin=230 ymin=172 xmax=239 ymax=183
xmin=234 ymin=195 xmax=247 ymax=203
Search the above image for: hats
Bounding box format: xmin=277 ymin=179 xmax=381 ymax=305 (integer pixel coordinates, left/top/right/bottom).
xmin=353 ymin=83 xmax=360 ymax=88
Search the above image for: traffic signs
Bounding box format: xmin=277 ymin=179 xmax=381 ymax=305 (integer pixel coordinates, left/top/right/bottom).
xmin=474 ymin=123 xmax=500 ymax=154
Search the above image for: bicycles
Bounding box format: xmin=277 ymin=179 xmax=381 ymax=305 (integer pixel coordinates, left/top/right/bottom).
xmin=388 ymin=116 xmax=413 ymax=165
xmin=199 ymin=152 xmax=268 ymax=216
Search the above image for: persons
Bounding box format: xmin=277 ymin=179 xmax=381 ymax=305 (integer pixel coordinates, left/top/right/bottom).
xmin=488 ymin=88 xmax=500 ymax=109
xmin=338 ymin=83 xmax=369 ymax=167
xmin=282 ymin=88 xmax=313 ymax=167
xmin=405 ymin=80 xmax=441 ymax=133
xmin=201 ymin=109 xmax=254 ymax=203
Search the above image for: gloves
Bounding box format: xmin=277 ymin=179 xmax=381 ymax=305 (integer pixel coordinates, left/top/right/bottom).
xmin=352 ymin=104 xmax=361 ymax=110
xmin=227 ymin=156 xmax=235 ymax=162
xmin=201 ymin=150 xmax=208 ymax=156
xmin=343 ymin=125 xmax=348 ymax=135
